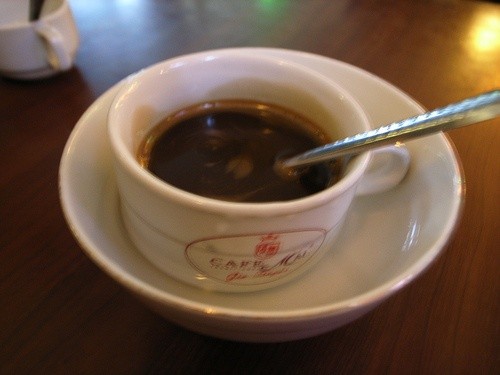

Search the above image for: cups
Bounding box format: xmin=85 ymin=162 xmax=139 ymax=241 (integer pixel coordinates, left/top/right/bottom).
xmin=0 ymin=0 xmax=78 ymax=80
xmin=106 ymin=49 xmax=410 ymax=294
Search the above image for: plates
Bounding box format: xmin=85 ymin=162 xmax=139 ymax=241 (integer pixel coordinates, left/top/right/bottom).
xmin=57 ymin=49 xmax=465 ymax=345
